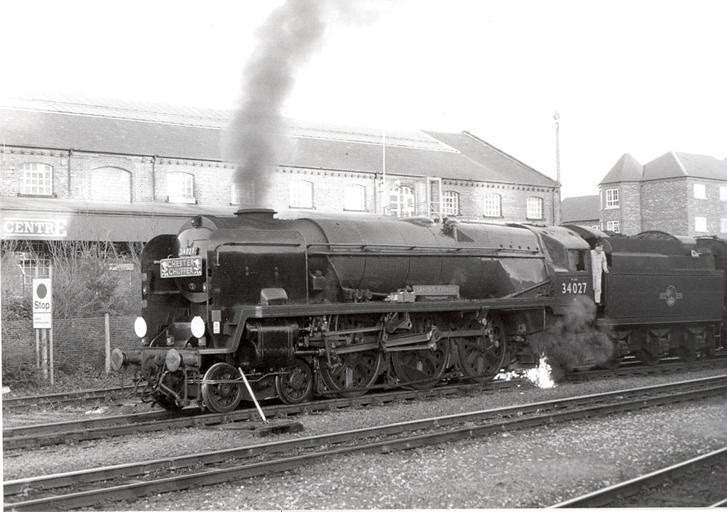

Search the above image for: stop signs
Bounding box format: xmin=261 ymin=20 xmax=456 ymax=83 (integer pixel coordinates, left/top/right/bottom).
xmin=33 ymin=279 xmax=53 ymax=328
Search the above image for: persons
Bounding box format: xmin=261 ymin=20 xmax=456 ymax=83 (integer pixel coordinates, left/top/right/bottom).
xmin=590 ymin=241 xmax=608 ymax=304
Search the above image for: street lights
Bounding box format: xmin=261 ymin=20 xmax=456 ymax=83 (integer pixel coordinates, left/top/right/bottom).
xmin=552 ymin=112 xmax=561 ymax=226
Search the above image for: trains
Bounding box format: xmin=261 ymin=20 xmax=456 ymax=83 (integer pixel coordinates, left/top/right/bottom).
xmin=111 ymin=208 xmax=727 ymax=414
